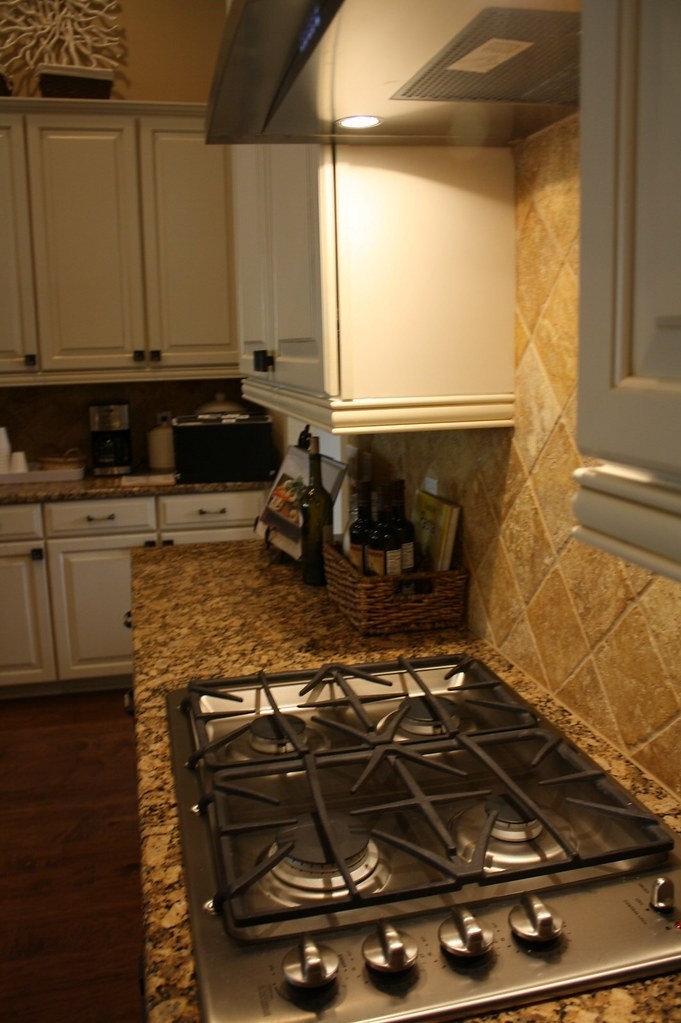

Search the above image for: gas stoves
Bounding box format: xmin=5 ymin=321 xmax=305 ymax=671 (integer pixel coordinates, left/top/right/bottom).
xmin=165 ymin=652 xmax=681 ymax=1023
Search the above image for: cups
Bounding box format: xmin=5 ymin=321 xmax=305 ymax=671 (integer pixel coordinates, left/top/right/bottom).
xmin=11 ymin=452 xmax=27 ymax=473
xmin=147 ymin=420 xmax=175 ymax=468
xmin=0 ymin=427 xmax=11 ymax=474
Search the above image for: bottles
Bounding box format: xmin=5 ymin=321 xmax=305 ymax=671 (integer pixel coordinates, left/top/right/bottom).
xmin=300 ymin=436 xmax=332 ymax=585
xmin=342 ymin=481 xmax=415 ymax=576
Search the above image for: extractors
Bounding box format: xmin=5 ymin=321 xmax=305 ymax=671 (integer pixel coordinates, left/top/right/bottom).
xmin=204 ymin=0 xmax=581 ymax=149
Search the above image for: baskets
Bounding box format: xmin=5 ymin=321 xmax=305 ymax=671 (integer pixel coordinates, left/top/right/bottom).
xmin=322 ymin=539 xmax=468 ymax=635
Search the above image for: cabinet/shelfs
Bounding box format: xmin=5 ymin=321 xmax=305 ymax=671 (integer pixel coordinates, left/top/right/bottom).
xmin=228 ymin=143 xmax=517 ymax=436
xmin=0 ymin=504 xmax=62 ymax=700
xmin=0 ymin=97 xmax=246 ymax=388
xmin=43 ymin=490 xmax=270 ymax=696
xmin=567 ymin=0 xmax=681 ymax=582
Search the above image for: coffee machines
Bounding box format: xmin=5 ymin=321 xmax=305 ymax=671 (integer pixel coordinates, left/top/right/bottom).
xmin=89 ymin=401 xmax=132 ymax=477
